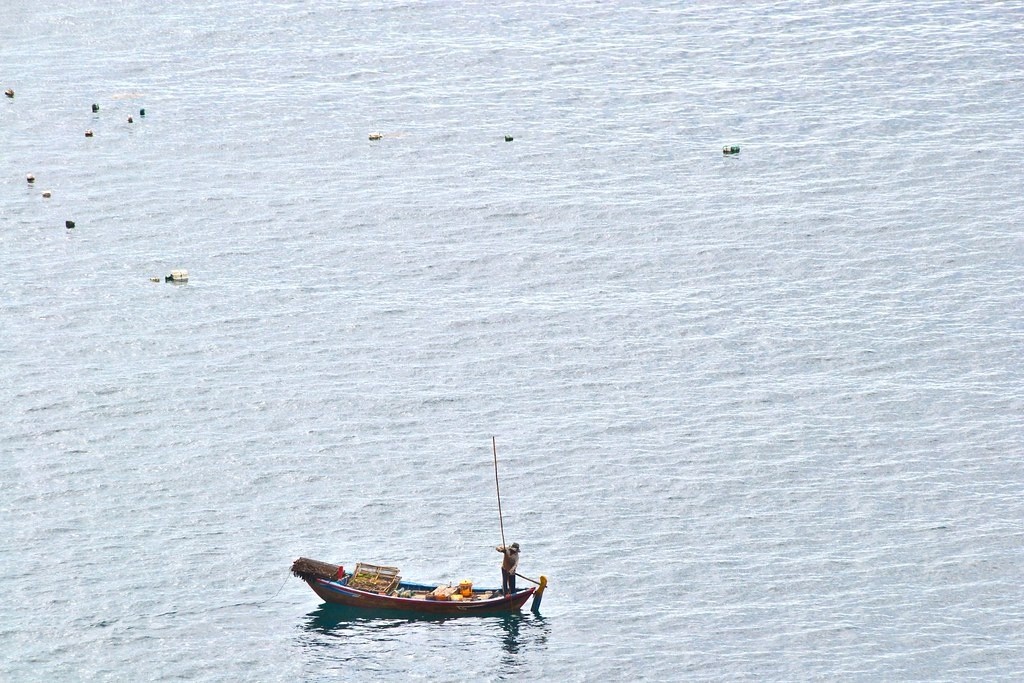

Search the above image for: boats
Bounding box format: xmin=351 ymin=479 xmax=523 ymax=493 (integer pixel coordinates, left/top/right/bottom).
xmin=288 ymin=554 xmax=537 ymax=624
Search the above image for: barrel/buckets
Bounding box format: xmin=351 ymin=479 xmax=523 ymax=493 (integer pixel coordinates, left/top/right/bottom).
xmin=459 ymin=580 xmax=473 ymax=597
xmin=452 ymin=594 xmax=464 ymax=601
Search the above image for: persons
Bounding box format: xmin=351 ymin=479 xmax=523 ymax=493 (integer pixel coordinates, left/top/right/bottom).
xmin=496 ymin=543 xmax=521 ymax=597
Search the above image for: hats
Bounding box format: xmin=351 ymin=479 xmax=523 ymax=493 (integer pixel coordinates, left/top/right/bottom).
xmin=509 ymin=543 xmax=522 ymax=553
